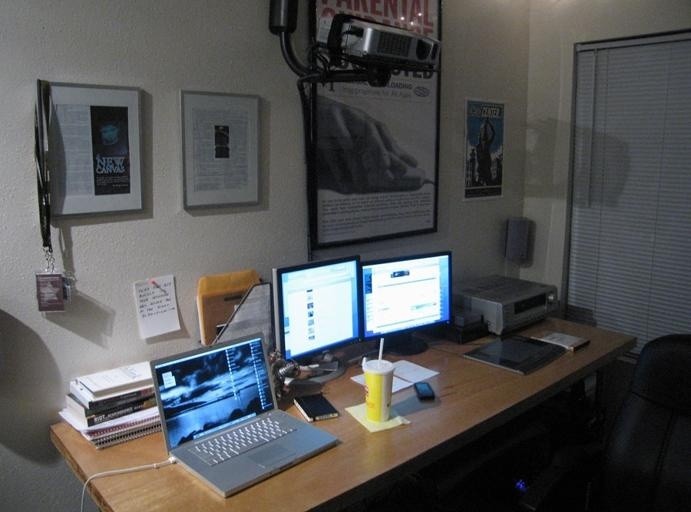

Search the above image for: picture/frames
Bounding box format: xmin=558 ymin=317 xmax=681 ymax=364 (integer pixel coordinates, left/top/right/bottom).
xmin=41 ymin=82 xmax=145 ymax=218
xmin=179 ymin=88 xmax=262 ymax=209
xmin=309 ymin=1 xmax=447 ymax=252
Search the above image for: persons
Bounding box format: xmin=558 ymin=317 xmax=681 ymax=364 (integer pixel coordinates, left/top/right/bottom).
xmin=94 ymin=117 xmax=129 ymax=158
xmin=311 ymin=92 xmax=418 ymax=196
xmin=470 ymin=116 xmax=503 ymax=185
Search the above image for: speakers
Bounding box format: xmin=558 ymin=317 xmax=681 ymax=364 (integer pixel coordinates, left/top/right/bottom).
xmin=503 ymin=217 xmax=530 ymax=265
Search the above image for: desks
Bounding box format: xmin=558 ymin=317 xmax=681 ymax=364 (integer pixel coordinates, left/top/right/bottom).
xmin=49 ymin=321 xmax=643 ymax=510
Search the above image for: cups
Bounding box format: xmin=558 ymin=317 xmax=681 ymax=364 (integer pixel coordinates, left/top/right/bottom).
xmin=363 ymin=359 xmax=396 ymax=423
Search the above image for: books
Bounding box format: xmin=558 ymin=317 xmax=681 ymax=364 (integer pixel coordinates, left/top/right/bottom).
xmin=294 ymin=393 xmax=339 ymax=423
xmin=534 ymin=329 xmax=591 ymax=353
xmin=56 ymin=359 xmax=165 ymax=451
xmin=462 ymin=332 xmax=568 ymax=377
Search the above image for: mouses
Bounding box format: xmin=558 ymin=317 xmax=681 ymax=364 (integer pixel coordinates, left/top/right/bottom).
xmin=414 ymin=382 xmax=436 ymax=401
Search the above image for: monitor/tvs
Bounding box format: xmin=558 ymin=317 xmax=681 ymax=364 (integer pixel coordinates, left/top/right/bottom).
xmin=359 ymin=250 xmax=453 ymax=356
xmin=272 ymin=254 xmax=361 ymax=383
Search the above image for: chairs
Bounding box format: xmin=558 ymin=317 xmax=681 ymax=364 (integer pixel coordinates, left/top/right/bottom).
xmin=512 ymin=330 xmax=691 ymax=511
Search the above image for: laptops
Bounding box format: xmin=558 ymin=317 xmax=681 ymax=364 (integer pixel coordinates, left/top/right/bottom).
xmin=149 ymin=332 xmax=340 ymax=499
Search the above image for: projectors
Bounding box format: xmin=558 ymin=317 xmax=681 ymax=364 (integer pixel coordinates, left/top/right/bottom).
xmin=316 ymin=15 xmax=442 ymax=66
